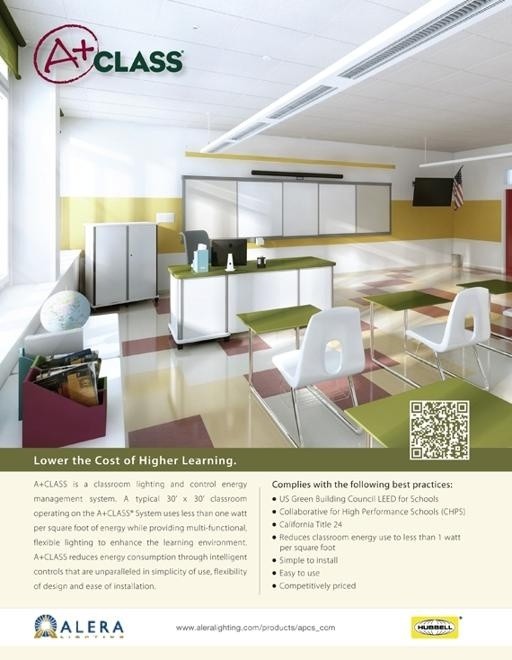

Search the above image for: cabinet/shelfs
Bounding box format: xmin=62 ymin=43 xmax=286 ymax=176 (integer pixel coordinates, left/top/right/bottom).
xmin=84 ymin=222 xmax=160 ymax=310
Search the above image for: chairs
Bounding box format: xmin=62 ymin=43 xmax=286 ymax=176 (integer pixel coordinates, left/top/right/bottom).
xmin=179 ymin=230 xmax=212 ymax=264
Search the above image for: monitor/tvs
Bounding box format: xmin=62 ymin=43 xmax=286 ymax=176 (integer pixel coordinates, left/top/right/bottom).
xmin=413 ymin=178 xmax=454 ymax=206
xmin=210 ymin=239 xmax=247 ymax=272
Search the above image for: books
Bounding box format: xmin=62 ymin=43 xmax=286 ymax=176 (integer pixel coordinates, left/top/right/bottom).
xmin=33 ymin=349 xmax=102 ymax=407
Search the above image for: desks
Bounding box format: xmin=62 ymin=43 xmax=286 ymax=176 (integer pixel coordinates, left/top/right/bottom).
xmin=235 ymin=278 xmax=512 ymax=449
xmin=166 ymin=256 xmax=337 ymax=349
xmin=0 ymin=312 xmax=128 ymax=450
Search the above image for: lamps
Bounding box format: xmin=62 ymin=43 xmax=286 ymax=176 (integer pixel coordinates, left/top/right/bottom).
xmin=199 ymin=1 xmax=512 ymax=157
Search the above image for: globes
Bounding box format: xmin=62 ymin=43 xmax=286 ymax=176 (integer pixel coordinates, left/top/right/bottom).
xmin=39 ymin=290 xmax=91 ymax=333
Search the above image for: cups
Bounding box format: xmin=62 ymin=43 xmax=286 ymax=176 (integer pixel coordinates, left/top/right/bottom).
xmin=257 ymin=257 xmax=267 ymax=268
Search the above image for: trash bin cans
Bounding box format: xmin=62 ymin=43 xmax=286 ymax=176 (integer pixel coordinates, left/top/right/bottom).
xmin=451 ymin=254 xmax=461 ymax=268
